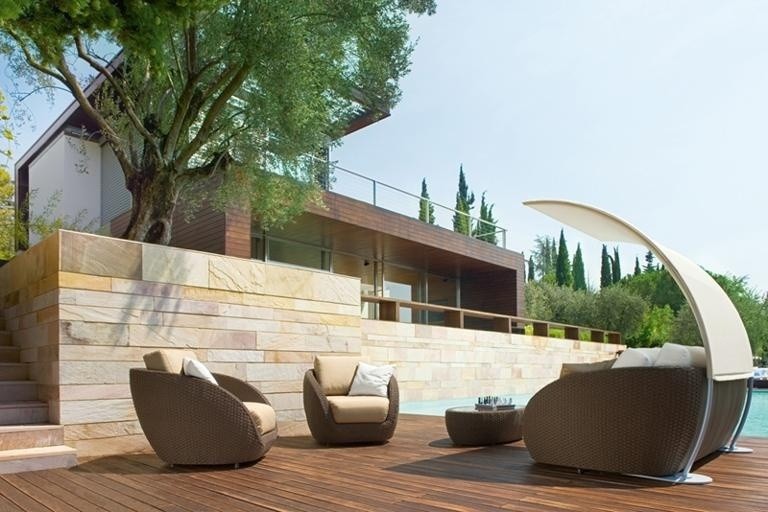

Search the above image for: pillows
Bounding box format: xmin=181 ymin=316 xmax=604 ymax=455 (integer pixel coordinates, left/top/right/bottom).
xmin=183 ymin=358 xmax=219 ymax=386
xmin=611 ymin=346 xmax=661 ymax=367
xmin=315 ymin=355 xmax=356 ymax=396
xmin=142 ymin=349 xmax=197 ymax=375
xmin=560 ymin=358 xmax=616 ymax=376
xmin=655 ymin=341 xmax=706 ymax=369
xmin=349 ymin=361 xmax=395 ymax=397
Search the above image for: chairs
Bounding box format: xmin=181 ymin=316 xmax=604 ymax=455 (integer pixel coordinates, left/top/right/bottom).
xmin=129 ymin=367 xmax=279 ymax=468
xmin=522 ymin=197 xmax=755 ymax=488
xmin=303 ymin=368 xmax=400 ymax=447
